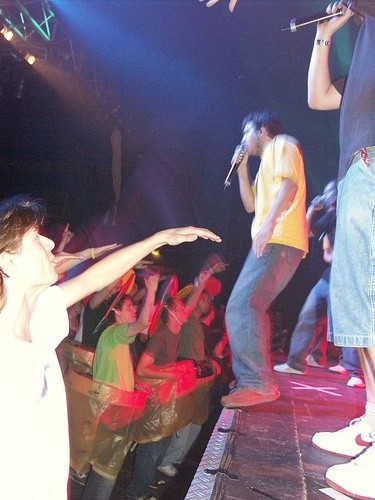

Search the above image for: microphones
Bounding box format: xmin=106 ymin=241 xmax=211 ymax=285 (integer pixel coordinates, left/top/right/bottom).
xmin=281 ymin=7 xmax=347 ymax=32
xmin=223 ymin=144 xmax=246 ymax=190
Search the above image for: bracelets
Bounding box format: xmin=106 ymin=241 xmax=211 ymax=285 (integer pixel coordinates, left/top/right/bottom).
xmin=313 ymin=39 xmax=332 ymax=46
xmin=92 ymin=247 xmax=96 ymax=260
xmin=210 ymin=268 xmax=215 ymax=274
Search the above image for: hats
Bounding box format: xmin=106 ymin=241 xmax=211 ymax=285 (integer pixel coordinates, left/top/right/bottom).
xmin=176 ymin=277 xmax=221 ymax=302
xmin=92 ymin=271 xmax=134 ymax=333
xmin=139 ymin=275 xmax=180 ymax=337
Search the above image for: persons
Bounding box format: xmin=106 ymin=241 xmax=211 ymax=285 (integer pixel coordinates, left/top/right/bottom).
xmin=0 ymin=106 xmax=375 ymax=500
xmin=195 ymin=0 xmax=375 ymax=500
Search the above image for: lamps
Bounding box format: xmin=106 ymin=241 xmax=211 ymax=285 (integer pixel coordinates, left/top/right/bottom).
xmin=19 ymin=48 xmax=36 ymax=65
xmin=0 ymin=21 xmax=14 ymax=41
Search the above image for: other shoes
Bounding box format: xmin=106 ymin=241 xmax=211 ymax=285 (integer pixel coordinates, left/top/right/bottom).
xmin=346 ymin=375 xmax=366 ymax=389
xmin=71 ymin=472 xmax=88 ymax=485
xmin=329 ymin=364 xmax=347 ymax=374
xmin=273 ymin=362 xmax=306 ymax=374
xmin=219 ymin=387 xmax=280 ymax=407
xmin=156 ymin=463 xmax=178 ymax=477
xmin=305 ymin=354 xmax=324 ymax=368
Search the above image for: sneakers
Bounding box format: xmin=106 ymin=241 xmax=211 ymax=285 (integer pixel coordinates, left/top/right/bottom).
xmin=325 ymin=440 xmax=375 ymax=500
xmin=311 ymin=412 xmax=375 ymax=460
